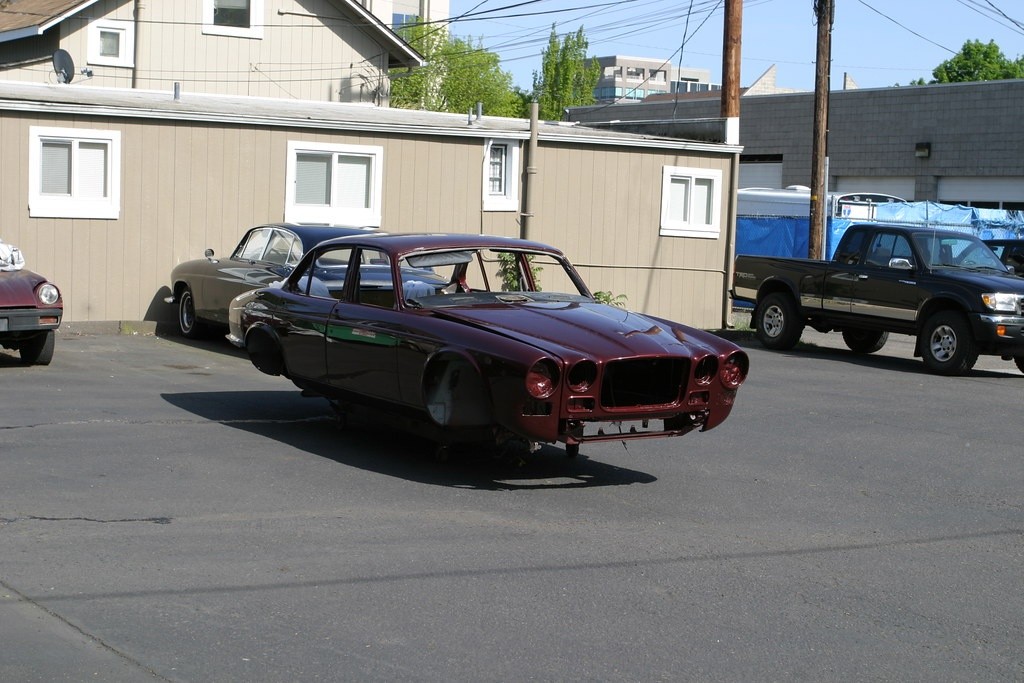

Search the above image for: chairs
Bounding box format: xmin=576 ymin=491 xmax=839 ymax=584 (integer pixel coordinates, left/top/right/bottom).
xmin=873 ymin=247 xmax=890 ymax=257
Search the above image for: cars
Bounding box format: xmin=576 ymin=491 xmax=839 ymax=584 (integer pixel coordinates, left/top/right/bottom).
xmin=224 ymin=233 xmax=749 ymax=447
xmin=162 ymin=223 xmax=450 ymax=336
xmin=0 ymin=265 xmax=65 ymax=365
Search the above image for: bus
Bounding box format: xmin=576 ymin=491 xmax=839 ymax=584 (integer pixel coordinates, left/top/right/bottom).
xmin=737 ymin=184 xmax=908 ymax=222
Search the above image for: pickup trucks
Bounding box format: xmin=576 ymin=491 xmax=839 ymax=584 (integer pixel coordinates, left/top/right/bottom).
xmin=732 ymin=223 xmax=1023 ymax=374
